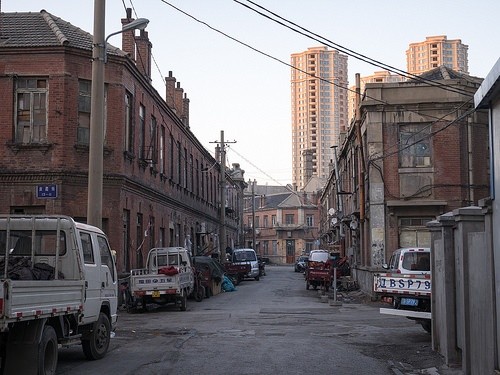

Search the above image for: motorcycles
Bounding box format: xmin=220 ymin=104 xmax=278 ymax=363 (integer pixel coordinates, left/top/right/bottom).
xmin=304 ymin=249 xmax=333 ymax=292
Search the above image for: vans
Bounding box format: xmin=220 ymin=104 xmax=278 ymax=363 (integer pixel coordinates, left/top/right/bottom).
xmin=231 ymin=248 xmax=259 ymax=281
xmin=381 ymin=247 xmax=430 ymax=274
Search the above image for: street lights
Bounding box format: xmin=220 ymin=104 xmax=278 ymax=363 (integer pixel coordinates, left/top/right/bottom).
xmin=86 ymin=0 xmax=149 ymax=231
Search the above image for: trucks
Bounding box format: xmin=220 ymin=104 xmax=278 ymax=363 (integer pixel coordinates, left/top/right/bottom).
xmin=0 ymin=212 xmax=119 ymax=375
xmin=129 ymin=246 xmax=195 ymax=311
xmin=373 ymin=272 xmax=432 ymax=333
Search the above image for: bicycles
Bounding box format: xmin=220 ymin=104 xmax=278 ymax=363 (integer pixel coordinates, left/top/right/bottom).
xmin=117 ymin=274 xmax=134 ymax=311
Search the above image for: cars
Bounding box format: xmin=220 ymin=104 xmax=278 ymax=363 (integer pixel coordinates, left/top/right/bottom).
xmin=294 ymin=255 xmax=308 ymax=272
xmin=192 ymin=255 xmax=231 ymax=297
